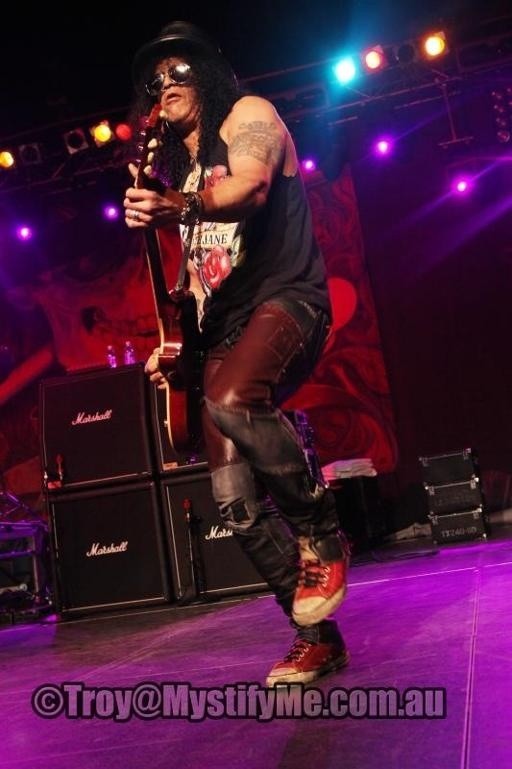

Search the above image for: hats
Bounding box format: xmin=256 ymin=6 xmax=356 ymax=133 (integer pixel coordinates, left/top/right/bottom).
xmin=128 ymin=18 xmax=243 ymax=102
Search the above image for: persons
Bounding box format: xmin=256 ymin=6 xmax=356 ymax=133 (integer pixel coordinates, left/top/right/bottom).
xmin=119 ymin=20 xmax=352 ymax=690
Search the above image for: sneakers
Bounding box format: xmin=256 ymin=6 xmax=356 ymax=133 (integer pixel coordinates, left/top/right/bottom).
xmin=264 ymin=634 xmax=354 ymax=690
xmin=286 ymin=527 xmax=353 ymax=630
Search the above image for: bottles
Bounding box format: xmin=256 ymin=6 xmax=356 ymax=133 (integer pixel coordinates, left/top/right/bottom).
xmin=54 ymin=452 xmax=66 ymax=483
xmin=120 ymin=339 xmax=134 ymax=367
xmin=106 ymin=342 xmax=121 ymax=369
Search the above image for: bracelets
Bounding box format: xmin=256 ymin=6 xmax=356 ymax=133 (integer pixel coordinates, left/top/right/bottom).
xmin=178 ymin=191 xmax=204 ymax=226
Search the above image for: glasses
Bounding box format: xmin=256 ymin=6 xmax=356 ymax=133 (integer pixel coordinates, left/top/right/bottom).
xmin=143 ymin=62 xmax=196 ymax=98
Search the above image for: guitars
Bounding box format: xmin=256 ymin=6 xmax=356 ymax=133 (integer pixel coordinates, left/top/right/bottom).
xmin=136 ymin=103 xmax=199 ymax=456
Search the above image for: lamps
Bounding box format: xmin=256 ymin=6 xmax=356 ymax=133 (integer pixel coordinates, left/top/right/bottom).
xmin=2 ymin=24 xmax=456 ymax=173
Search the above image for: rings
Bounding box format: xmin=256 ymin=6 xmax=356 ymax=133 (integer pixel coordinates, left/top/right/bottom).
xmin=132 ymin=210 xmax=140 ymax=220
